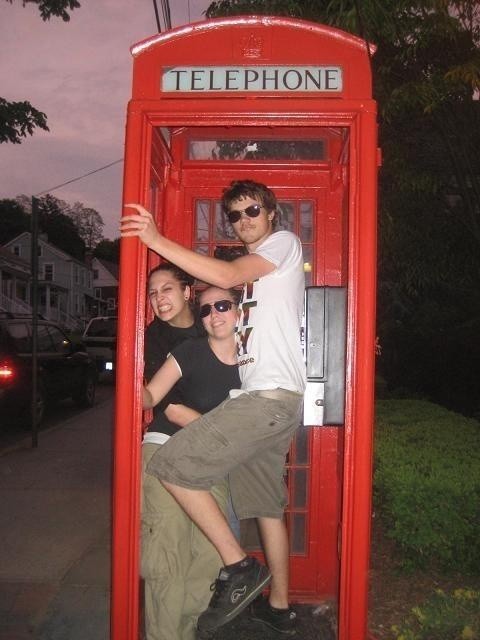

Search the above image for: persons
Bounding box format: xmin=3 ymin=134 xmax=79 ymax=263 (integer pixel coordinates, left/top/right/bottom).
xmin=118 ymin=181 xmax=306 ymax=634
xmin=141 ymin=286 xmax=241 ymax=640
xmin=144 ymin=262 xmax=208 ymax=427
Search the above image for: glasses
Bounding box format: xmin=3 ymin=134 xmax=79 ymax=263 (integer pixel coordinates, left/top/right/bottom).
xmin=229 ymin=205 xmax=265 ymax=223
xmin=199 ymin=300 xmax=238 ymax=318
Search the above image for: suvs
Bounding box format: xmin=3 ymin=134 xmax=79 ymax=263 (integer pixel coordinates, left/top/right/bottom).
xmin=0 ymin=312 xmax=100 ymax=434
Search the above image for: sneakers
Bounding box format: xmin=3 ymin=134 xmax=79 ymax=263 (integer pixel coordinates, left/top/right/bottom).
xmin=198 ymin=557 xmax=272 ymax=632
xmin=238 ymin=593 xmax=298 ymax=634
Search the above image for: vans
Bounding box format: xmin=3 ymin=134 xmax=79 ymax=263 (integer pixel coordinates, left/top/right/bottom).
xmin=80 ymin=315 xmax=118 ymax=385
